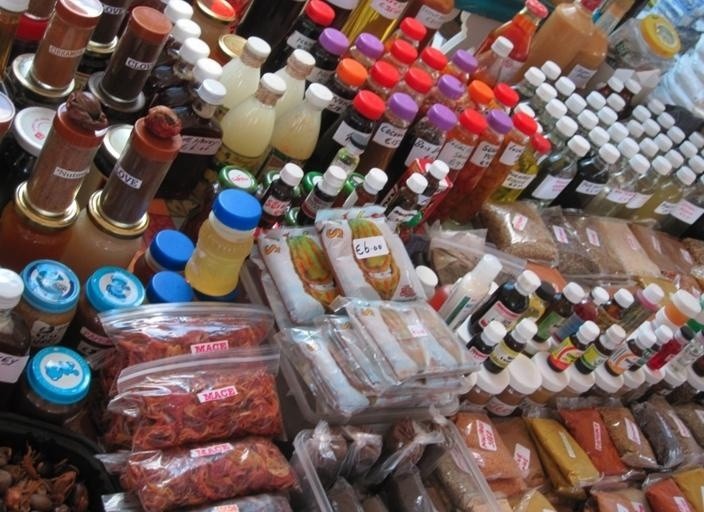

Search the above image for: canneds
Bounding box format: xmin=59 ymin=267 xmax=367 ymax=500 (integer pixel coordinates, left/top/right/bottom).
xmin=17 ymin=259 xmax=145 ymax=430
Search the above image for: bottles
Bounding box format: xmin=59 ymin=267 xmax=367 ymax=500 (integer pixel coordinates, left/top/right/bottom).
xmin=0 ymin=0 xmax=704 ymax=419
